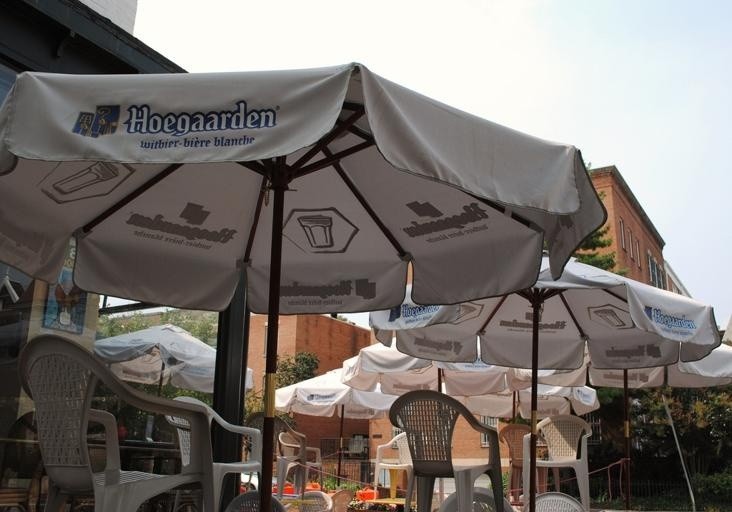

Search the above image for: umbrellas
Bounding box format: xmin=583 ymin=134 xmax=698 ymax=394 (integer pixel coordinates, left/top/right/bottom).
xmin=367 ymin=249 xmax=720 ymax=511
xmin=274 ymin=366 xmax=400 ymax=489
xmin=94 ymin=322 xmax=255 ymax=443
xmin=506 ymin=339 xmax=732 ymax=510
xmin=341 ymin=336 xmax=590 ymax=417
xmin=438 ymin=379 xmax=600 ymax=426
xmin=0 ymin=62 xmax=609 ymax=511
